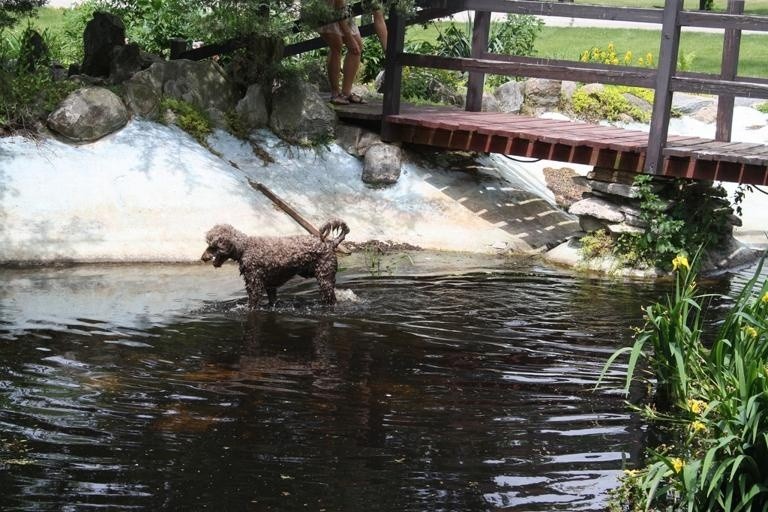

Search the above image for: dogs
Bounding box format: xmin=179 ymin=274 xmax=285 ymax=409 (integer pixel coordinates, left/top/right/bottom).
xmin=201 ymin=218 xmax=350 ymax=312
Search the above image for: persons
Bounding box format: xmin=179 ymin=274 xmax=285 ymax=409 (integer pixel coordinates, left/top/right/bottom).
xmin=314 ymin=0 xmax=388 ymax=105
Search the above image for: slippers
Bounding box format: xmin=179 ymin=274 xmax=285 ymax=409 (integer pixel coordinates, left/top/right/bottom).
xmin=347 ymin=93 xmax=367 ymax=104
xmin=329 ymin=94 xmax=350 ymax=105
